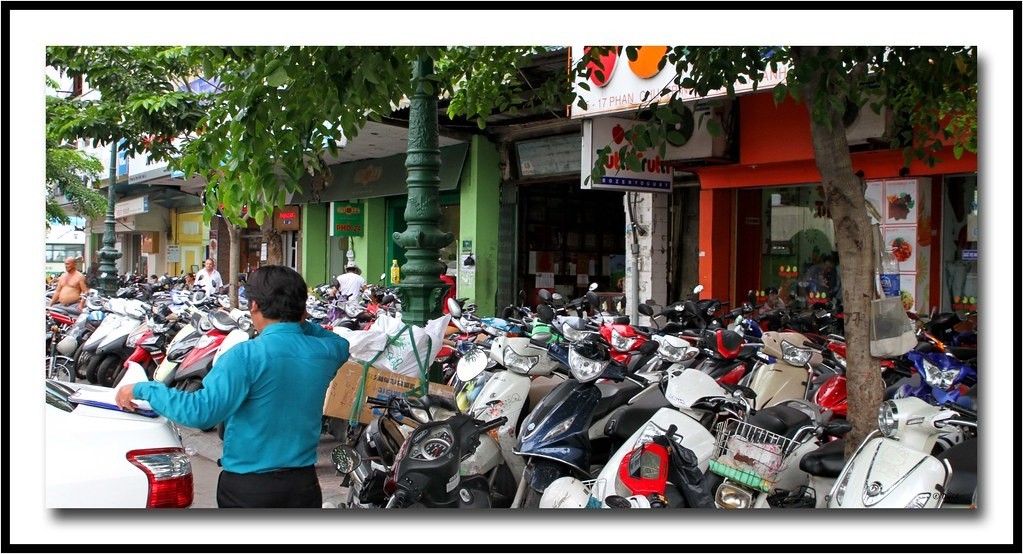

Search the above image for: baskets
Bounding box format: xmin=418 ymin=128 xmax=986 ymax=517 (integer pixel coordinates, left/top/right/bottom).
xmin=710 ymin=417 xmax=801 ymax=494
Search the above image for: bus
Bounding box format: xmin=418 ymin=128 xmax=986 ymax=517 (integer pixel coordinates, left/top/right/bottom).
xmin=46 ymin=242 xmax=87 ymax=276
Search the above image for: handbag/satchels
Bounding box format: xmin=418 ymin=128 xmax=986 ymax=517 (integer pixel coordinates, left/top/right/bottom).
xmin=869 ymin=296 xmax=917 ymax=357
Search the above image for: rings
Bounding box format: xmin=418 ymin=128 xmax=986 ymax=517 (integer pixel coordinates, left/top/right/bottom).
xmin=122 ymin=400 xmax=126 ymax=403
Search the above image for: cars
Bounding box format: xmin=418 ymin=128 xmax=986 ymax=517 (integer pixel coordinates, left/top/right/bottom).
xmin=46 ymin=378 xmax=195 ymax=509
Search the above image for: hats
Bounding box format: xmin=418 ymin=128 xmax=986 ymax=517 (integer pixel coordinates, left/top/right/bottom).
xmin=344 ymin=262 xmax=361 ymax=275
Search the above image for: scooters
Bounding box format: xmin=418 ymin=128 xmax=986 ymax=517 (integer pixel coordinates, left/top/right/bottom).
xmin=46 ymin=270 xmax=978 ymax=509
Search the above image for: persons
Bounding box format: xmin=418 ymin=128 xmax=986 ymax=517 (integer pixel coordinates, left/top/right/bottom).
xmin=758 ymin=287 xmax=779 ymax=314
xmin=328 ymin=261 xmax=367 ymax=304
xmin=185 ymin=258 xmax=247 ymax=299
xmin=115 ymin=265 xmax=350 ymax=508
xmin=116 ymin=271 xmax=131 ymax=281
xmin=804 ymin=257 xmax=844 ymax=306
xmin=49 ymin=256 xmax=90 ymax=312
xmin=150 ymin=274 xmax=169 ymax=291
xmin=617 ymin=277 xmax=625 ymax=289
xmin=438 ymin=261 xmax=455 ymax=314
xmin=92 ymin=250 xmax=99 ymax=263
